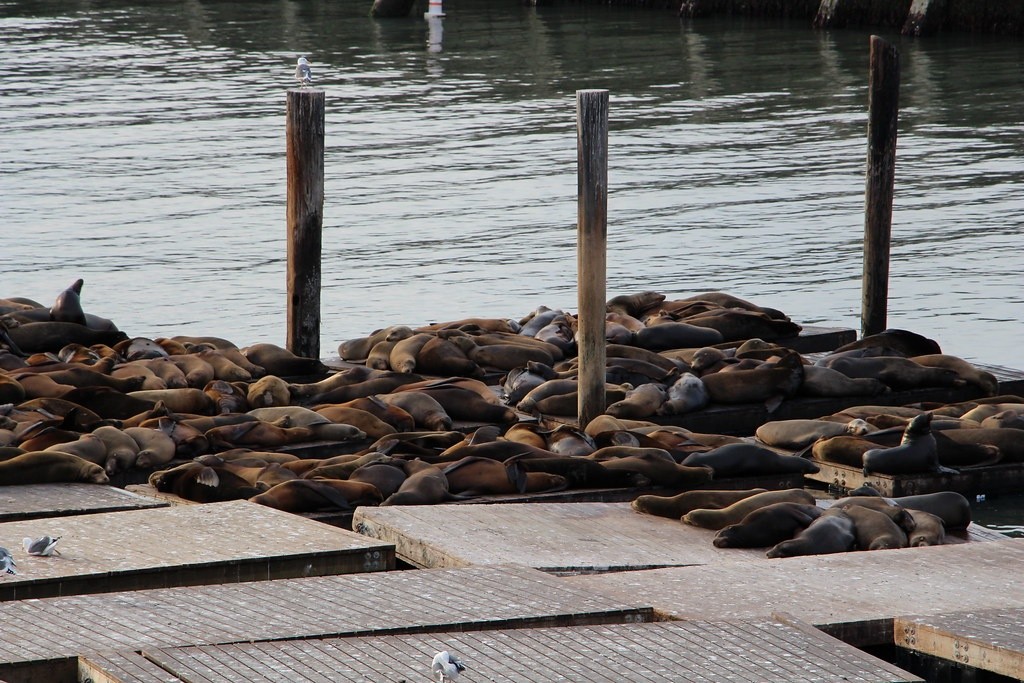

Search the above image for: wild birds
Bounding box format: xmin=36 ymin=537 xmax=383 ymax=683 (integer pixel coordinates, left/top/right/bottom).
xmin=21 ymin=536 xmax=64 ymax=556
xmin=431 ymin=651 xmax=466 ymax=683
xmin=0 ymin=547 xmax=17 ymax=577
